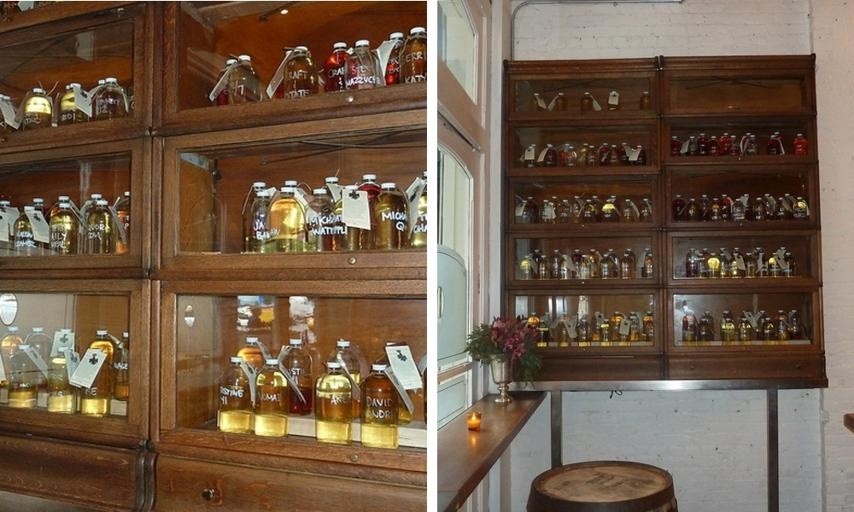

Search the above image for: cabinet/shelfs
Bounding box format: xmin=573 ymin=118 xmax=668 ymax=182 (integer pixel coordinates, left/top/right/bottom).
xmin=0 ymin=0 xmax=436 ymax=512
xmin=500 ymin=56 xmax=826 ymax=390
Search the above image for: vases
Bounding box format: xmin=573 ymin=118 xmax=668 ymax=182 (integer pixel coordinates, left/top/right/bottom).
xmin=483 ymin=356 xmax=514 ymax=403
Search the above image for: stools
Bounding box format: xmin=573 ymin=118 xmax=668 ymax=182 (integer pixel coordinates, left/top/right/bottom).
xmin=522 ymin=459 xmax=679 ymax=512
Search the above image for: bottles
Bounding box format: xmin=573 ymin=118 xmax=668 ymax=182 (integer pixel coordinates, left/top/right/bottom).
xmin=55 ymin=196 xmax=70 ymax=211
xmin=559 ymin=200 xmax=571 ymax=223
xmin=409 ymin=171 xmax=427 ymax=251
xmin=767 ymin=136 xmax=779 ymax=155
xmin=744 ymin=253 xmax=755 ymax=277
xmin=687 ymin=137 xmax=697 ymax=156
xmin=46 ymin=329 xmax=76 ymax=415
xmin=699 ymin=195 xmax=710 ymax=222
xmin=682 ymin=312 xmax=696 ymax=342
xmin=590 ymin=196 xmax=601 ymax=223
xmin=732 ymin=199 xmax=744 ymax=220
xmin=795 ymin=134 xmax=806 ymax=156
xmin=228 ymin=56 xmax=259 ymax=105
xmin=747 ymin=135 xmax=758 ymax=155
xmin=788 ymin=309 xmax=800 ymax=325
xmin=0 ymin=327 xmax=24 ymax=384
xmin=216 ymin=357 xmax=251 ymax=436
xmin=58 ymin=83 xmax=90 ymax=125
xmin=561 ymin=144 xmax=571 ymax=166
xmin=550 ymin=250 xmax=562 ymax=279
xmin=565 ymin=147 xmax=577 ymax=167
xmin=346 ymin=40 xmax=374 ymax=92
xmin=0 ymin=94 xmax=15 ymax=132
xmin=609 ymin=196 xmax=617 ymax=208
xmin=384 ymin=32 xmax=404 ymax=86
xmin=703 ymin=312 xmax=714 ymax=341
xmin=609 ymin=145 xmax=619 ymax=165
xmin=762 ymin=318 xmax=775 ymax=341
xmin=696 ymin=254 xmax=708 ymax=278
xmin=702 ymin=247 xmax=708 ymax=258
xmin=673 ymin=195 xmax=684 ymax=221
xmin=606 ymin=249 xmax=619 ymax=278
xmin=696 ymin=134 xmax=707 ymax=156
xmin=777 ymin=309 xmax=787 ymax=332
xmin=282 ymin=181 xmax=306 ymax=199
xmin=525 ymin=145 xmax=536 ymax=167
xmin=708 ymin=136 xmax=719 ymax=156
xmin=742 ymin=194 xmax=749 ymax=218
xmin=361 ymin=363 xmax=398 ymax=450
xmin=570 ymin=196 xmax=583 ymax=222
xmin=706 ymin=253 xmax=720 ymax=278
xmin=27 ymin=327 xmax=52 ymax=388
xmin=687 ymin=248 xmax=697 ymax=277
xmin=328 ymin=342 xmax=362 ymax=419
xmin=550 ymin=196 xmax=558 ymax=222
xmin=624 ymin=248 xmax=636 ymax=273
xmin=773 ymin=131 xmax=785 ymax=154
xmin=306 ymin=189 xmax=332 ymax=252
xmin=719 ymin=133 xmax=729 ymax=155
xmin=577 ymin=143 xmax=589 ymax=165
xmin=719 ymin=248 xmax=725 ymax=256
xmin=544 ymin=145 xmax=556 ymax=167
xmin=267 ymin=187 xmax=305 ymax=254
xmin=698 ymin=318 xmax=711 ymax=341
xmin=80 ymin=351 xmax=108 ymax=416
xmin=764 ymin=194 xmax=774 ymax=221
xmin=721 ymin=194 xmax=731 ymax=221
xmin=626 ymin=312 xmax=639 ymax=342
xmin=634 ymin=146 xmax=645 ymax=165
xmin=28 ymin=198 xmax=46 ymax=223
xmin=589 ymin=249 xmax=599 ymax=277
xmin=710 ymin=198 xmax=722 ymax=221
xmin=114 ymin=332 xmax=128 ymax=401
xmin=540 ymin=200 xmax=549 ymax=224
xmin=613 ymin=312 xmax=621 ymax=342
xmin=730 ymin=135 xmax=738 ymax=156
xmin=357 ymin=174 xmax=380 ymax=250
xmin=517 ymin=201 xmax=527 ymax=223
xmin=400 ymin=28 xmax=426 ymax=84
xmin=521 ymin=255 xmax=532 ymax=281
xmin=777 ymin=315 xmax=789 ymax=341
xmin=754 ymin=198 xmax=766 ymax=221
xmin=90 ymin=330 xmax=115 ymax=396
xmin=757 ymin=310 xmax=767 ymax=340
xmin=784 ymin=193 xmax=792 ymax=219
xmin=14 ymin=206 xmax=41 ymax=258
xmin=535 ymin=92 xmax=547 ymax=112
xmin=640 ymin=198 xmax=651 ymax=223
xmin=373 ymin=342 xmax=413 ymax=423
xmin=732 ymin=248 xmax=741 ymax=254
xmin=572 ymin=249 xmax=582 ymax=278
xmin=96 ymin=77 xmax=124 ymax=120
xmin=555 ymin=313 xmax=568 ymax=343
xmin=720 ymin=309 xmax=733 ymax=340
xmin=373 ymin=183 xmax=407 ymax=250
xmin=719 ymin=253 xmax=729 ymax=278
xmin=783 ymin=253 xmax=795 ymax=276
xmin=600 ymin=254 xmax=612 ymax=279
xmin=639 ymin=92 xmax=650 ymax=112
xmin=593 ymin=311 xmax=602 ymax=341
xmin=607 ymin=87 xmax=620 ymax=111
xmin=757 ymin=252 xmax=768 ymax=277
xmin=620 ymin=254 xmax=632 ymax=280
xmin=0 ymin=200 xmax=14 ymax=251
xmin=602 ymin=319 xmax=610 ymax=342
xmin=620 ymin=198 xmax=634 ymax=223
xmin=753 ymin=247 xmax=767 ymax=277
xmin=528 ymin=312 xmax=539 ymax=330
xmin=333 ymin=185 xmax=358 ymax=252
xmin=599 ymin=143 xmax=609 ymax=166
xmin=722 ymin=319 xmax=735 ymax=341
xmin=251 ymin=191 xmax=271 ymax=255
xmin=620 ymin=142 xmax=631 ymax=166
xmin=278 ymin=339 xmax=312 ymax=415
xmin=240 ymin=181 xmax=266 ymax=255
xmin=583 ymin=200 xmax=595 ymax=223
xmin=580 ymin=92 xmax=592 ymax=112
xmin=531 ymin=250 xmax=541 ymax=278
xmin=46 ymin=203 xmax=77 ymax=255
xmin=22 ymin=88 xmax=51 ymax=129
xmin=644 ymin=253 xmax=654 ymax=277
xmin=587 ymin=146 xmax=596 ymax=166
xmin=641 ymin=248 xmax=650 ymax=278
xmin=325 ymin=42 xmax=351 ymax=92
xmin=643 ymin=311 xmax=653 ymax=342
xmin=559 ymin=254 xmax=572 ymax=279
xmin=738 ymin=318 xmax=752 ymax=342
xmin=602 ymin=200 xmax=616 ymax=222
xmin=554 ymin=93 xmax=567 ymax=114
xmin=536 ymin=320 xmax=548 ymax=348
xmin=729 ymin=252 xmax=744 ymax=278
xmin=419 ymin=353 xmax=427 ymax=431
xmin=81 ymin=194 xmax=101 ymax=221
xmin=775 ymin=198 xmax=786 ymax=220
xmin=579 ymin=254 xmax=590 ymax=279
xmin=671 ymin=137 xmax=680 ymax=157
xmin=793 ymin=197 xmax=808 ymax=221
xmin=316 ymin=364 xmax=350 ymax=445
xmin=324 ymin=177 xmax=339 ymax=204
xmin=255 ymin=358 xmax=286 ymax=438
xmin=577 ymin=318 xmax=590 ymax=342
xmin=743 ymin=133 xmax=752 ymax=152
xmin=769 ymin=253 xmax=782 ymax=276
xmin=787 ymin=319 xmax=802 ymax=341
xmin=539 ymin=255 xmax=550 ymax=280
xmin=217 ymin=59 xmax=239 ymax=105
xmin=113 ymin=192 xmax=131 ymax=257
xmin=238 ymin=338 xmax=269 ymax=375
xmin=284 ymin=46 xmax=315 ymax=99
xmin=527 ymin=197 xmax=538 ymax=224
xmin=6 ymin=345 xmax=37 ymax=408
xmin=687 ymin=198 xmax=698 ymax=221
xmin=85 ymin=200 xmax=111 ymax=255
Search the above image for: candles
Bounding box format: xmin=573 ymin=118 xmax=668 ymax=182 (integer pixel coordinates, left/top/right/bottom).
xmin=467 ymin=410 xmax=482 ymax=432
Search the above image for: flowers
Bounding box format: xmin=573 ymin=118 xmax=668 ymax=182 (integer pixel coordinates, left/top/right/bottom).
xmin=464 ymin=302 xmax=540 ymax=390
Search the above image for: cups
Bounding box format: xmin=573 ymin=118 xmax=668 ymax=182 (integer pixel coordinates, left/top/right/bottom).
xmin=467 ymin=412 xmax=481 ymax=430
